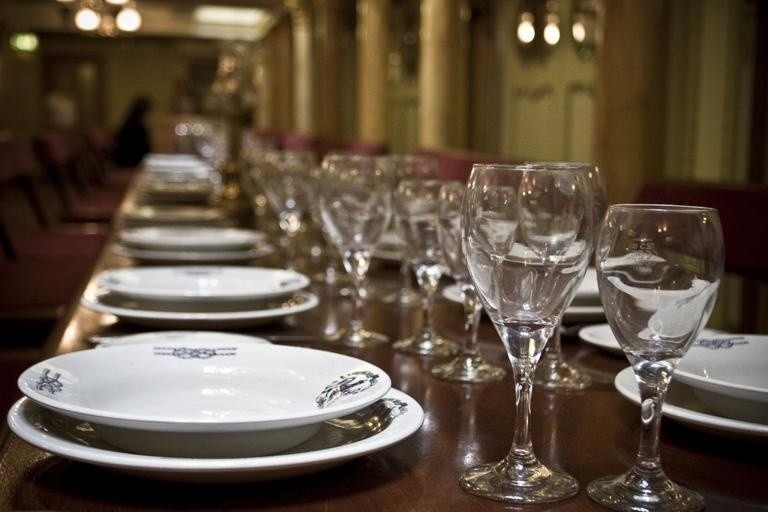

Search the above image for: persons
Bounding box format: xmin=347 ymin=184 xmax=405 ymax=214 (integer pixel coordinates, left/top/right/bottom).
xmin=113 ymin=94 xmax=155 ymax=169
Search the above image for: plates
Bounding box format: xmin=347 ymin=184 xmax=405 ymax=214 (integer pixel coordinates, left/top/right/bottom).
xmin=440 ymin=269 xmax=768 ymax=435
xmin=110 ymin=223 xmax=276 ymax=266
xmin=5 ymin=331 xmax=424 ymax=475
xmin=79 ymin=262 xmax=321 ymax=328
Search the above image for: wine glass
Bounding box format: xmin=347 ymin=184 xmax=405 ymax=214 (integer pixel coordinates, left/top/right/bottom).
xmin=238 ymin=143 xmax=727 ymax=512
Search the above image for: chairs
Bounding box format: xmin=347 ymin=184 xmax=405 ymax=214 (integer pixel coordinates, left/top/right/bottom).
xmin=1 ymin=127 xmax=133 ymax=321
